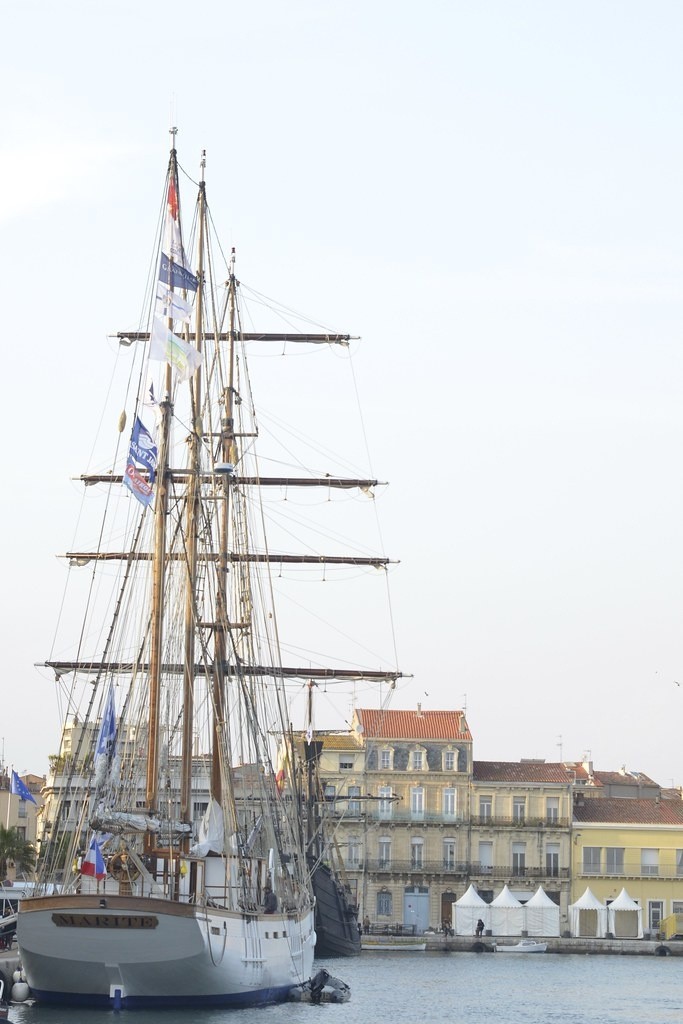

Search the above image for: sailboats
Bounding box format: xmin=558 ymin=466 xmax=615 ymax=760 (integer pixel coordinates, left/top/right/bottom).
xmin=14 ymin=122 xmax=414 ymax=1014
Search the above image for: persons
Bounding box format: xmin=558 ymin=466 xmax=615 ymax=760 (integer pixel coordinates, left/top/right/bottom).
xmin=475 ymin=919 xmax=484 ymax=938
xmin=363 ymin=915 xmax=371 ymax=935
xmin=263 ymin=886 xmax=279 ymax=915
xmin=1 ymin=908 xmax=17 ymax=951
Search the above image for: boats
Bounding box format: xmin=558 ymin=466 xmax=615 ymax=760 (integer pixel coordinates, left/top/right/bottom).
xmin=361 ymin=940 xmax=426 ymax=951
xmin=494 ymin=939 xmax=547 ymax=953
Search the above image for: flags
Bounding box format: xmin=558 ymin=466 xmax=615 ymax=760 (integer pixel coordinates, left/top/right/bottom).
xmin=119 ymin=172 xmax=204 ymax=506
xmin=11 ymin=770 xmax=37 ymax=805
xmin=80 ymin=838 xmax=107 ymax=882
xmin=97 ymin=688 xmax=117 ymax=757
